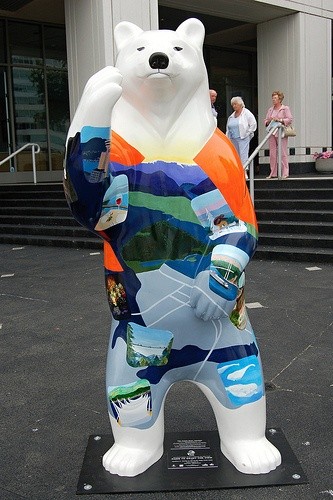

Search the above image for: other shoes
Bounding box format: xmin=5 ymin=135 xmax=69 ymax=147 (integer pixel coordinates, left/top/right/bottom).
xmin=245 ymin=171 xmax=248 ymax=180
xmin=282 ymin=176 xmax=288 ymax=179
xmin=266 ymin=176 xmax=276 ymax=179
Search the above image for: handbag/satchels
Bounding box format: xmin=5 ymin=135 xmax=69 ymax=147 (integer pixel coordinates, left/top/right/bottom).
xmin=282 ymin=123 xmax=296 ymax=137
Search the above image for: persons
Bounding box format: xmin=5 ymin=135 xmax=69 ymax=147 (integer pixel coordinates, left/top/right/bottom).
xmin=264 ymin=90 xmax=294 ymax=179
xmin=208 ymin=90 xmax=218 ymax=127
xmin=225 ymin=96 xmax=257 ymax=179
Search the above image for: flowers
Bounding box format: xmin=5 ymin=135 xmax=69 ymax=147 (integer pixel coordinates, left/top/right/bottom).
xmin=312 ymin=150 xmax=333 ymax=159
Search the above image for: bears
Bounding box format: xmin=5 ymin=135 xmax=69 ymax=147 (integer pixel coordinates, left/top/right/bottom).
xmin=61 ymin=17 xmax=283 ymax=478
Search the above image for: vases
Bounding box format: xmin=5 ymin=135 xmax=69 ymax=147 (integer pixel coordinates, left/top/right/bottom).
xmin=315 ymin=158 xmax=333 ymax=174
